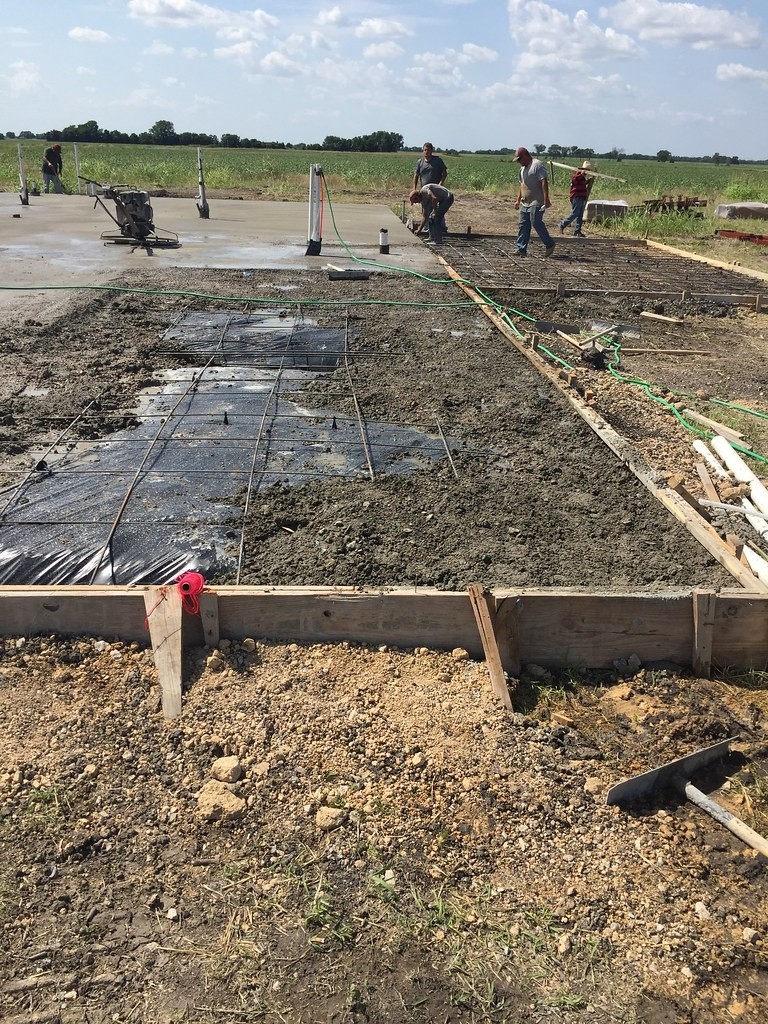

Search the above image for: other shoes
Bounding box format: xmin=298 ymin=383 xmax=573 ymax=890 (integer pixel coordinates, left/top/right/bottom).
xmin=423 ymin=239 xmax=435 ymax=242
xmin=442 ymin=230 xmax=447 ymax=233
xmin=574 ymin=232 xmax=585 ymax=237
xmin=508 ymin=251 xmax=526 ymax=256
xmin=427 ymin=242 xmax=443 ymax=246
xmin=424 ymin=229 xmax=429 ymax=233
xmin=545 ymin=243 xmax=556 ymax=257
xmin=559 ymin=224 xmax=564 ymax=235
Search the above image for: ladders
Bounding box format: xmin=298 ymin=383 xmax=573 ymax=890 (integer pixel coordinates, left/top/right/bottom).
xmin=714 ymin=230 xmax=768 ymax=251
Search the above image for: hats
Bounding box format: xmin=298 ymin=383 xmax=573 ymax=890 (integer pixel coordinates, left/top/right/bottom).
xmin=578 ymin=161 xmax=591 ymax=171
xmin=513 ymin=146 xmax=528 ymax=162
xmin=410 ymin=190 xmax=418 ymax=206
xmin=53 ymin=144 xmax=62 ymax=153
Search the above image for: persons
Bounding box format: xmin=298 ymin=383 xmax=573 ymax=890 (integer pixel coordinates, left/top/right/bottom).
xmin=509 ymin=147 xmax=556 ymax=258
xmin=559 ymin=161 xmax=595 ymax=238
xmin=41 ymin=144 xmax=64 ymax=194
xmin=409 ymin=183 xmax=454 ymax=246
xmin=413 ymin=142 xmax=447 ymax=231
